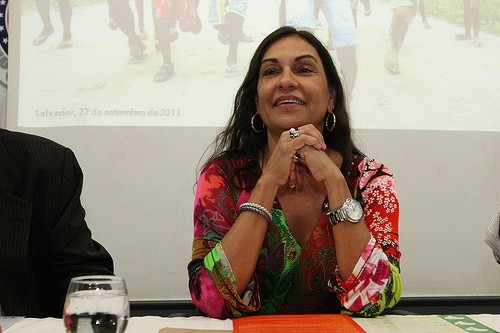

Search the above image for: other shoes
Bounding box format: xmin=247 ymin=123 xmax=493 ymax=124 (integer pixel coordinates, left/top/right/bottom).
xmin=218 ymin=33 xmax=230 ymax=46
xmin=473 ymin=39 xmax=482 ymax=48
xmin=59 ymin=29 xmax=73 ymax=49
xmin=455 ymin=33 xmax=471 ymax=40
xmin=223 ymin=63 xmax=239 ymax=77
xmin=384 ymin=50 xmax=399 ymax=74
xmin=154 ymin=63 xmax=174 ymax=82
xmin=127 ymin=46 xmax=146 ymax=64
xmin=191 ymin=10 xmax=201 ymax=35
xmin=32 ymin=27 xmax=54 ymax=46
xmin=107 ymin=20 xmax=117 ymax=31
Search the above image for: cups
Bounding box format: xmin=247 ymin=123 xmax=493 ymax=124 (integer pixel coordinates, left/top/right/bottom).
xmin=62 ymin=275 xmax=130 ymax=333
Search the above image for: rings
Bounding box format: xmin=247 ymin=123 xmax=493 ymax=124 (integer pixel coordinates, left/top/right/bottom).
xmin=293 ymin=152 xmax=301 ymax=160
xmin=289 ymin=127 xmax=299 ymax=141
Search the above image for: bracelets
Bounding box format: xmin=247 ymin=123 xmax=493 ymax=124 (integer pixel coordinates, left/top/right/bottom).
xmin=236 ymin=202 xmax=272 ymax=224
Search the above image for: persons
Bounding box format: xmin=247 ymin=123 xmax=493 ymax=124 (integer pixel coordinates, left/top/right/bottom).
xmin=151 ymin=0 xmax=250 ymax=82
xmin=458 ymin=0 xmax=480 ymax=47
xmin=187 ymin=27 xmax=401 ymax=317
xmin=0 ymin=127 xmax=115 ymax=318
xmin=384 ymin=0 xmax=433 ymax=73
xmin=282 ymin=0 xmax=371 ymax=102
xmin=33 ymin=0 xmax=73 ymax=48
xmin=107 ymin=0 xmax=148 ymax=64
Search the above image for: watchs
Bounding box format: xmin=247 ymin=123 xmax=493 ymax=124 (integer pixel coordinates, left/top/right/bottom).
xmin=326 ymin=198 xmax=363 ymax=225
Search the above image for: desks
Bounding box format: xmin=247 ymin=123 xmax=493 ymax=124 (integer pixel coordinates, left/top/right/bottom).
xmin=0 ymin=313 xmax=500 ymax=333
